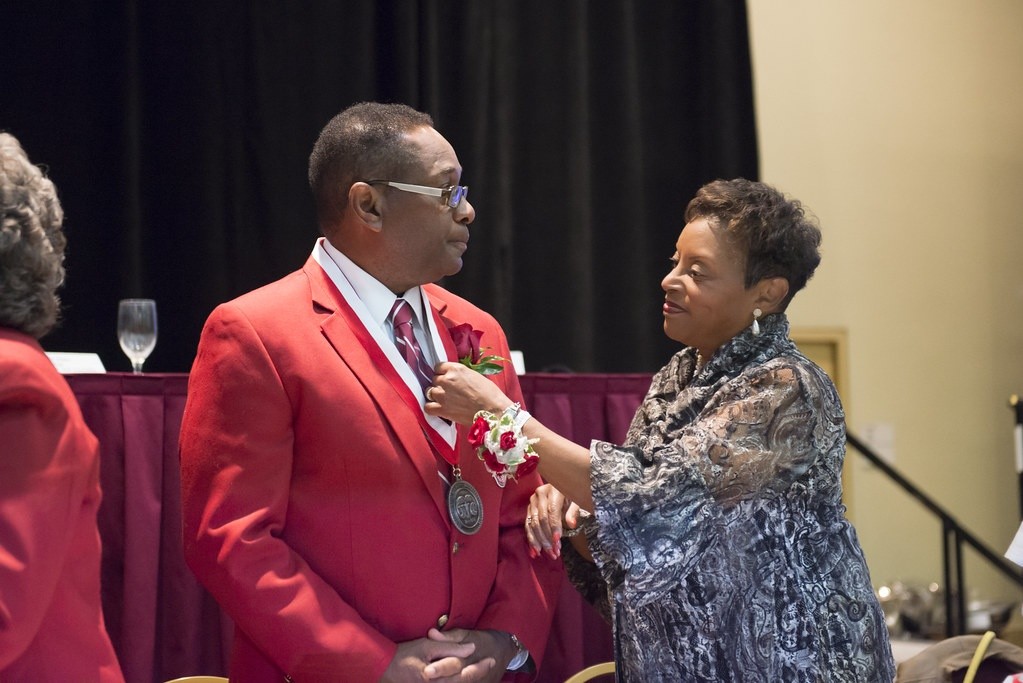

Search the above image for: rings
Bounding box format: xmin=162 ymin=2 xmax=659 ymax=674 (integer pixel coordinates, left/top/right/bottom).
xmin=526 ymin=513 xmax=538 ymax=523
xmin=425 ymin=387 xmax=435 ymax=401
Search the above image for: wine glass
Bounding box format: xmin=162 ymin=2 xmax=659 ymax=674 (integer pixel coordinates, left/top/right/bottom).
xmin=118 ymin=300 xmax=157 ymax=375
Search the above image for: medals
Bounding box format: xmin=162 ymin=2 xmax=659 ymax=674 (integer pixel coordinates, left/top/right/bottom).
xmin=448 ymin=480 xmax=484 ymax=536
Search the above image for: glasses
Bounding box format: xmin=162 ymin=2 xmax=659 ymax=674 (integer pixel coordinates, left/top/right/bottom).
xmin=366 ymin=180 xmax=468 ymax=209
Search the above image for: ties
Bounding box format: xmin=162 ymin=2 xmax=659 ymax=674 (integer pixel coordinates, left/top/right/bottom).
xmin=385 ymin=299 xmax=453 ymax=427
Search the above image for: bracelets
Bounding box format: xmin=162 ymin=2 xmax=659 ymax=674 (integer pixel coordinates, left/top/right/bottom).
xmin=509 ymin=634 xmax=522 ymax=655
xmin=563 ymin=523 xmax=584 ymax=538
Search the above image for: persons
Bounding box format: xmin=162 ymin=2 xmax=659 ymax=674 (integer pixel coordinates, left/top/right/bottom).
xmin=422 ymin=179 xmax=896 ymax=682
xmin=178 ymin=101 xmax=562 ymax=683
xmin=0 ymin=132 xmax=128 ymax=683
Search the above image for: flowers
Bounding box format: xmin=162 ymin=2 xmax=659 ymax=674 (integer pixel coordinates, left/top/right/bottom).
xmin=466 ymin=410 xmax=540 ymax=486
xmin=446 ymin=319 xmax=511 ymax=375
